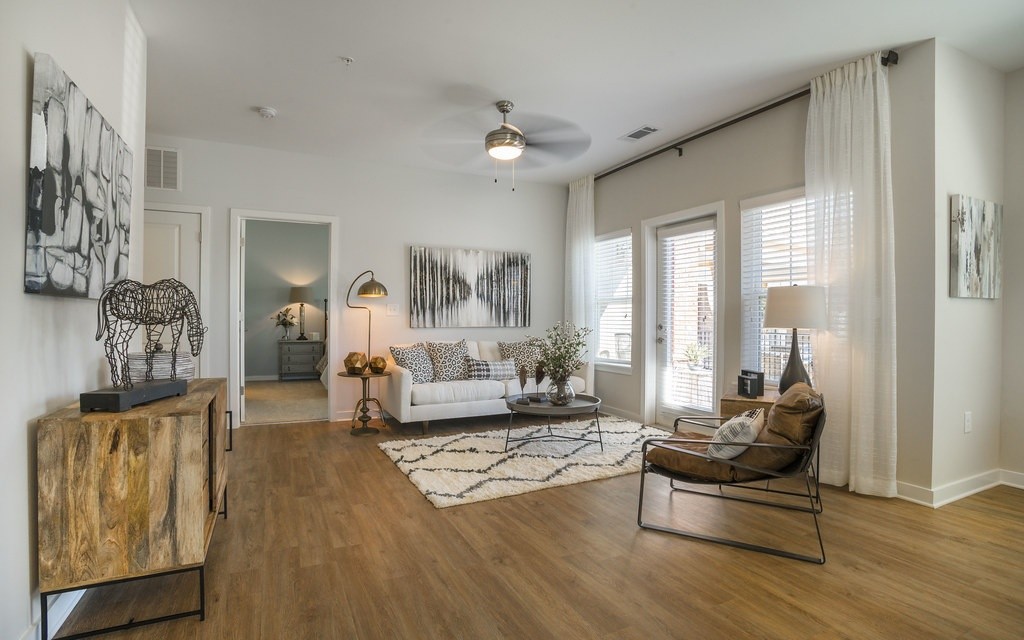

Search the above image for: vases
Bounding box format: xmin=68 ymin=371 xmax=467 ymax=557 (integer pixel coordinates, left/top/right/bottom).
xmin=545 ymin=378 xmax=576 ymax=405
xmin=285 ymin=326 xmax=290 ymax=340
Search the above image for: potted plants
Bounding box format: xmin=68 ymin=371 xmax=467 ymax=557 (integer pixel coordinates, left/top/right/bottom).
xmin=683 ymin=342 xmax=711 ymax=370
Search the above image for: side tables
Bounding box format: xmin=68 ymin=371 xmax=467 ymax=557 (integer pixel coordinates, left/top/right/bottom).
xmin=338 ymin=371 xmax=391 ymax=437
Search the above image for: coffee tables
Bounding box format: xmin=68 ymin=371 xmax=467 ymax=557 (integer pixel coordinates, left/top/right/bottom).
xmin=505 ymin=393 xmax=604 ymax=455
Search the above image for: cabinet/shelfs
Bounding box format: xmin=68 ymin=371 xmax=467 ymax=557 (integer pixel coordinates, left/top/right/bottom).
xmin=719 ymin=385 xmax=781 ymax=489
xmin=37 ymin=377 xmax=233 ymax=640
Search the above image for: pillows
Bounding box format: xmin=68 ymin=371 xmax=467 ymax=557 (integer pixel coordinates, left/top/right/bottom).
xmin=464 ymin=356 xmax=518 ymax=381
xmin=425 ymin=339 xmax=472 ymax=381
xmin=389 ymin=342 xmax=435 ymax=384
xmin=707 ymin=405 xmax=765 ymax=464
xmin=497 ymin=340 xmax=547 ymax=377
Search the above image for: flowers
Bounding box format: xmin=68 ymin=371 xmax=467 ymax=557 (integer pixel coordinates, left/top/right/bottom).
xmin=271 ymin=307 xmax=300 ymax=328
xmin=525 ymin=320 xmax=594 ymax=381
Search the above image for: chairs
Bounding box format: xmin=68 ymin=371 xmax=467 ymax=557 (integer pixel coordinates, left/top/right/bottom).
xmin=638 ymin=380 xmax=825 ymax=566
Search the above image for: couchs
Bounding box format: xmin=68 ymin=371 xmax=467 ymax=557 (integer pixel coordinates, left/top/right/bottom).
xmin=378 ymin=340 xmax=594 ymax=436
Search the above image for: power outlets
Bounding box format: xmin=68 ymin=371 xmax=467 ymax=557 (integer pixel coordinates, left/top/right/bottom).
xmin=965 ymin=412 xmax=973 ymax=434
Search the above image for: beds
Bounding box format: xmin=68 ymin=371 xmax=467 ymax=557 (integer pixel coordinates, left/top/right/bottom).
xmin=316 ymin=299 xmax=328 ymax=390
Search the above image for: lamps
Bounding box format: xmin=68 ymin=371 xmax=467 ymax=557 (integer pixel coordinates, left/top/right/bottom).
xmin=762 ymin=283 xmax=828 ymax=396
xmin=346 ymin=269 xmax=389 ymax=428
xmin=484 ymin=134 xmax=527 ymax=161
xmin=289 ymin=287 xmax=314 ymax=340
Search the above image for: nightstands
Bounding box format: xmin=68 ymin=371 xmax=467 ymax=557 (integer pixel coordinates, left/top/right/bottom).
xmin=277 ymin=340 xmax=324 ymax=384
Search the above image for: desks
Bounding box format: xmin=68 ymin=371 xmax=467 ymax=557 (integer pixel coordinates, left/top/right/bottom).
xmin=675 ymin=370 xmax=713 ymax=404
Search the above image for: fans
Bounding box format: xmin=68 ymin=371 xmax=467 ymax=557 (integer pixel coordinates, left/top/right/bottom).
xmin=420 ymin=100 xmax=593 ymax=177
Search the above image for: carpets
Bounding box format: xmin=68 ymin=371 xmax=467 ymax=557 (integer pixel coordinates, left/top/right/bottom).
xmin=377 ymin=413 xmax=672 ymax=509
